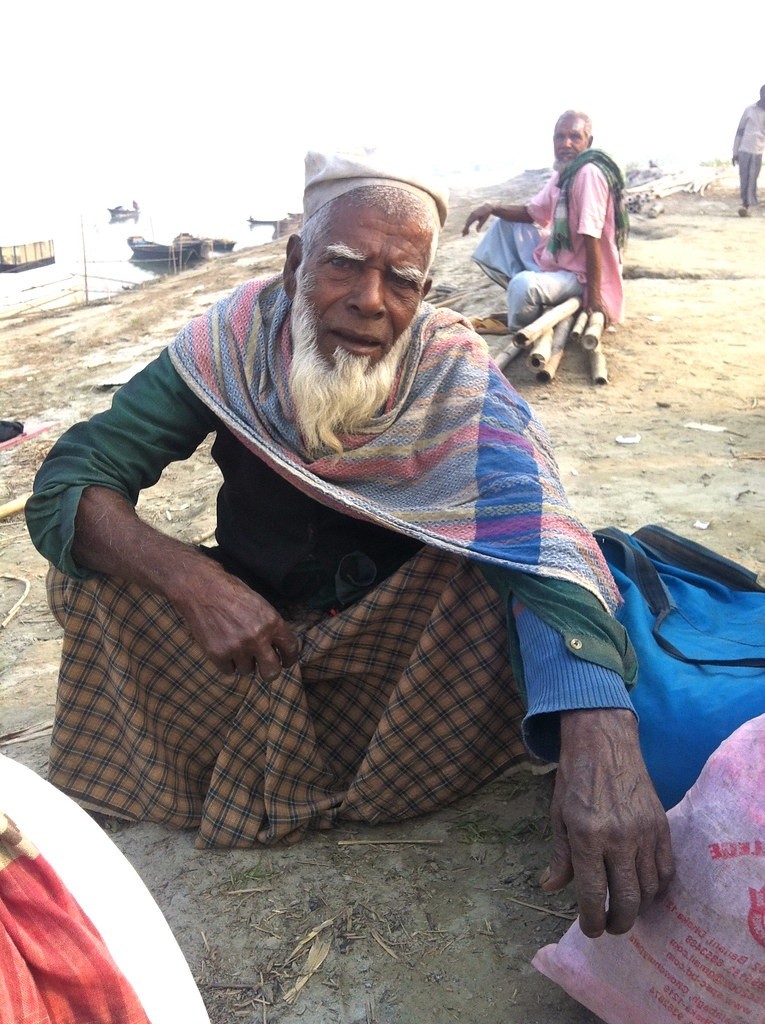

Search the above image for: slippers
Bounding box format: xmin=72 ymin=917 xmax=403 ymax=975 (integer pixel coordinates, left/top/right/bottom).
xmin=470 ymin=318 xmax=510 ymax=335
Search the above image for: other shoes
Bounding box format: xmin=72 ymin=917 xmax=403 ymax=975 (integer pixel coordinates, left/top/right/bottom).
xmin=738 ymin=207 xmax=750 ymax=217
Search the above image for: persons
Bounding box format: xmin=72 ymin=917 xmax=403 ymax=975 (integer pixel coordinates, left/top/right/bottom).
xmin=731 ymin=83 xmax=765 ymax=217
xmin=25 ymin=153 xmax=679 ymax=938
xmin=462 ymin=111 xmax=629 ymax=335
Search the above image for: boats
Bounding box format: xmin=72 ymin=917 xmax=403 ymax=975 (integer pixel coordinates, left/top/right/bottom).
xmin=108 ymin=200 xmax=140 ymax=218
xmin=173 ymin=233 xmax=237 ymax=252
xmin=125 ymin=235 xmax=193 ymax=267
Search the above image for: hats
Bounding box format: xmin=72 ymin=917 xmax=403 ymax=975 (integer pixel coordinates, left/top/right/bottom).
xmin=302 ymin=152 xmax=450 ymax=225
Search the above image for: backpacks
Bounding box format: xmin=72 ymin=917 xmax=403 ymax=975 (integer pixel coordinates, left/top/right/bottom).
xmin=591 ymin=525 xmax=765 ymax=805
